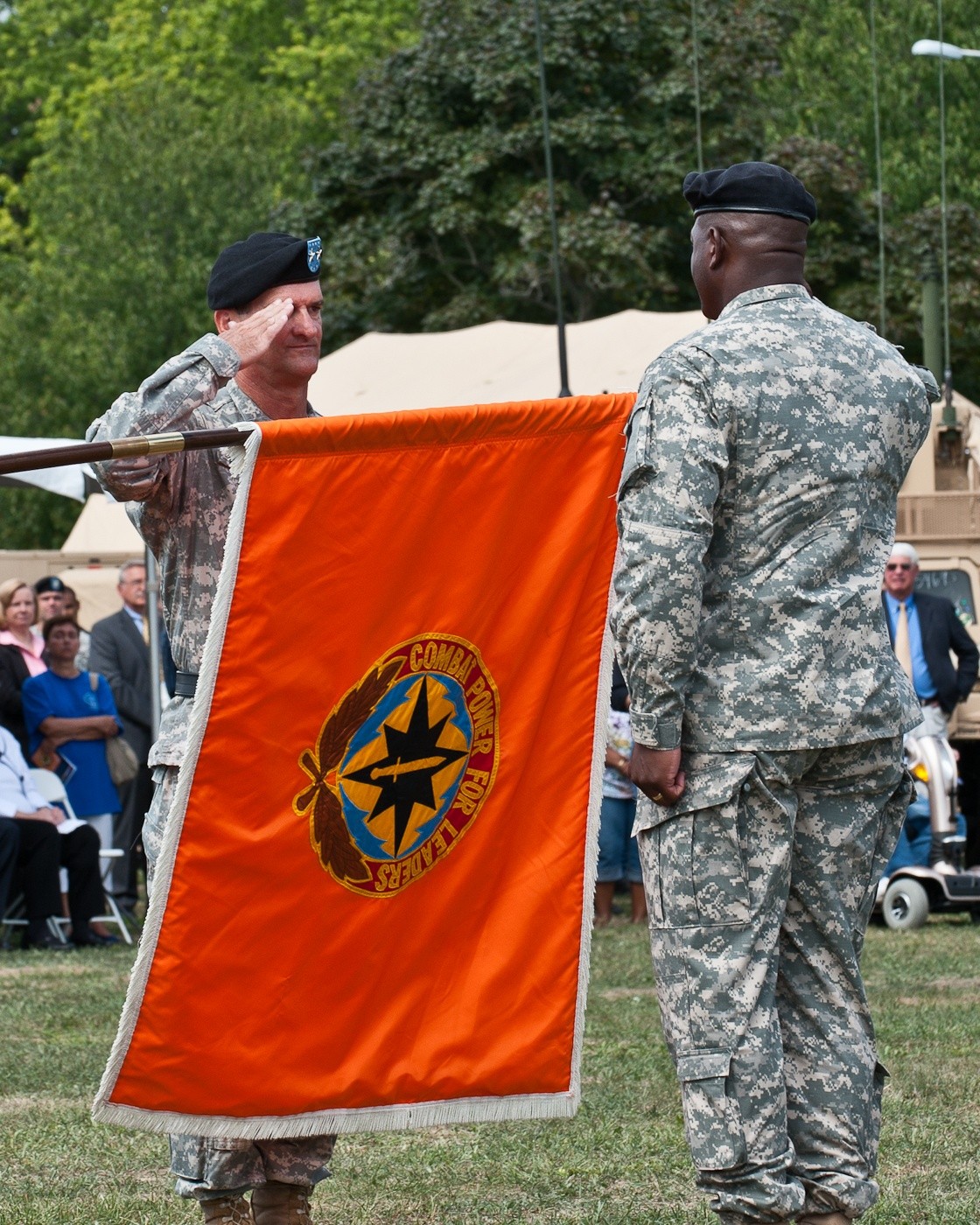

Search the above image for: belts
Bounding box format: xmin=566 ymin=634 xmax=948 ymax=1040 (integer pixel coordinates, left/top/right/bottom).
xmin=174 ymin=670 xmax=200 ymax=696
xmin=918 ymin=695 xmax=937 ymax=707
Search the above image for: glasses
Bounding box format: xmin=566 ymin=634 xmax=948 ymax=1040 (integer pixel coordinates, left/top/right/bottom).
xmin=886 ymin=563 xmax=913 ymax=570
xmin=121 ymin=580 xmax=144 ymax=587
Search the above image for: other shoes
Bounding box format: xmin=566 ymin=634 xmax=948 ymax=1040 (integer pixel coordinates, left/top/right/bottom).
xmin=68 ymin=927 xmax=122 ymax=949
xmin=200 ymin=1196 xmax=256 ymax=1225
xmin=249 ymin=1186 xmax=312 ymax=1225
xmin=21 ymin=932 xmax=76 ymax=951
xmin=110 ymin=908 xmax=136 ymax=920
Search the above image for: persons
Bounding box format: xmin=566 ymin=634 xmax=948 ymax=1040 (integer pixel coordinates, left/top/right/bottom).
xmin=616 ymin=163 xmax=939 ymax=1225
xmin=873 ymin=543 xmax=980 ymax=902
xmin=0 ymin=560 xmax=173 ymax=951
xmin=594 ymin=681 xmax=648 ymax=928
xmin=89 ymin=230 xmax=340 ymax=1225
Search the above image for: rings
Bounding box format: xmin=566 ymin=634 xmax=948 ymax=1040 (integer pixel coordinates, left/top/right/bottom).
xmin=652 ymin=793 xmax=662 ymax=801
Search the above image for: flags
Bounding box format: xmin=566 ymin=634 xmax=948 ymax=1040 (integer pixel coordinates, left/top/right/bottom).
xmin=90 ymin=395 xmax=636 ymax=1141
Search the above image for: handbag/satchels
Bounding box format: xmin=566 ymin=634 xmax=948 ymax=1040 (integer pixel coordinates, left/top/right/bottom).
xmin=90 ymin=673 xmax=139 ymax=786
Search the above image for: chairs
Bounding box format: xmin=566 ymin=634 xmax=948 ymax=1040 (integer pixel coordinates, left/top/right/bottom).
xmin=0 ymin=768 xmax=133 ymax=947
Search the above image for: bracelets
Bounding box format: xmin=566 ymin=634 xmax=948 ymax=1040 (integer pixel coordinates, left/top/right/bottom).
xmin=615 ymin=757 xmax=626 ymax=769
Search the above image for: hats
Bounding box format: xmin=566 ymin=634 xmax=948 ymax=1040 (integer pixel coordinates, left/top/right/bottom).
xmin=683 ymin=162 xmax=817 ymax=226
xmin=207 ymin=232 xmax=323 ymax=309
xmin=33 ymin=577 xmax=64 ymax=591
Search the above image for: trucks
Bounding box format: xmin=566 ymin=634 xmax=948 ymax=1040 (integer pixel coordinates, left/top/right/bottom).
xmin=879 ymin=492 xmax=979 ymax=738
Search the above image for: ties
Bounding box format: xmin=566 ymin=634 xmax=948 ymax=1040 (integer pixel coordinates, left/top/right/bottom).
xmin=142 ymin=615 xmax=150 ymax=647
xmin=895 ymin=603 xmax=913 ymax=685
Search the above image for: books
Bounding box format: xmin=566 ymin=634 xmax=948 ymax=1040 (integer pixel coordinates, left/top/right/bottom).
xmin=29 ymin=740 xmax=77 ymax=788
